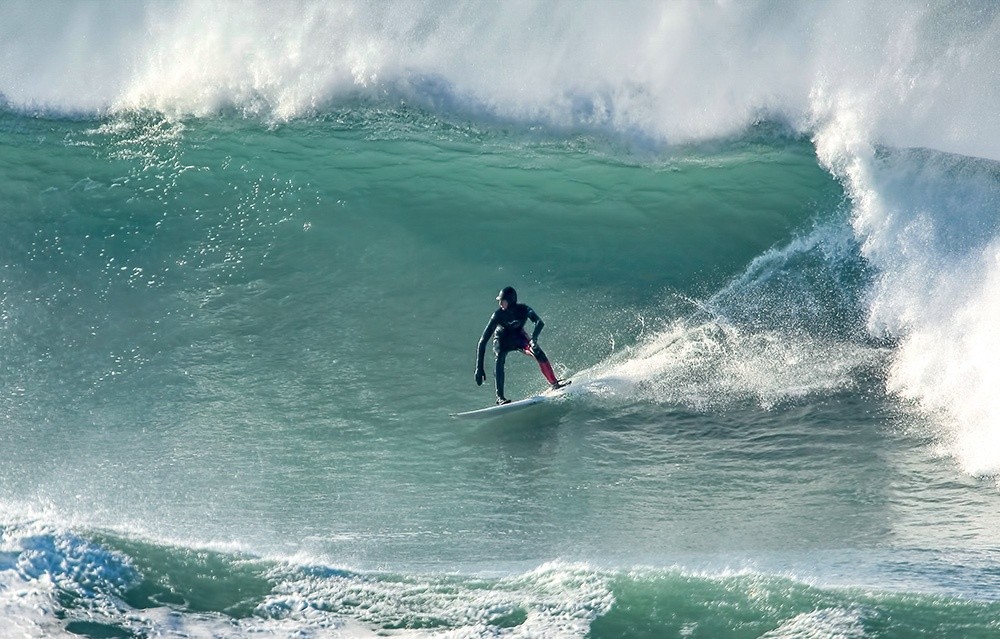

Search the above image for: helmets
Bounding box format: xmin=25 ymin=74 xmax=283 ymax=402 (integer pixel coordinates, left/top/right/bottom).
xmin=496 ymin=286 xmax=516 ymax=302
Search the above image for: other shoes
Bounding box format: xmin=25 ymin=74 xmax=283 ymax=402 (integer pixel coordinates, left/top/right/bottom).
xmin=552 ymin=380 xmax=571 ymax=390
xmin=496 ymin=397 xmax=511 ymax=405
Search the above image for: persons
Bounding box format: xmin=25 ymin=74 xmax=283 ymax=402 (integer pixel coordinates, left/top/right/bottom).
xmin=474 ymin=287 xmax=571 ymax=405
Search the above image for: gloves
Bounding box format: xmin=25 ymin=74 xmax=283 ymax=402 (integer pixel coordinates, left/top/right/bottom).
xmin=474 ymin=369 xmax=486 ymax=386
xmin=528 ymin=338 xmax=537 ymax=349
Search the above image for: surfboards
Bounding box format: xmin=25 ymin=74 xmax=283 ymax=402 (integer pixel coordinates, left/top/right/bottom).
xmin=448 ymin=394 xmax=565 ymax=419
